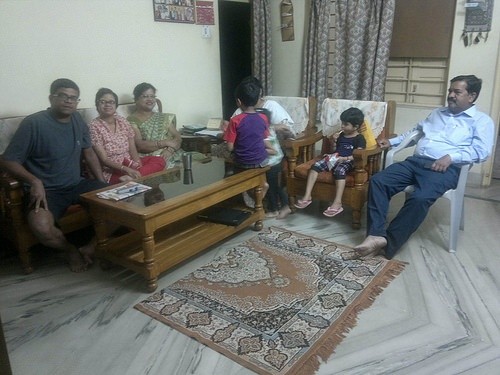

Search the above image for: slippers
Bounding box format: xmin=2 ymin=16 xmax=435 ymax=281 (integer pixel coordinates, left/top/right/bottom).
xmin=294 ymin=199 xmax=313 ymax=209
xmin=322 ymin=206 xmax=343 ymax=217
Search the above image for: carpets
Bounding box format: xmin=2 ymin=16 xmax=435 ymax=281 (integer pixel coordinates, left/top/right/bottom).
xmin=133 ymin=225 xmax=410 ymax=375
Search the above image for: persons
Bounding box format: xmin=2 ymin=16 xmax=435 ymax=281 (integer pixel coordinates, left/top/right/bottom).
xmin=255 ymin=108 xmax=291 ymax=218
xmin=230 ymin=78 xmax=295 ymax=139
xmin=294 ymin=107 xmax=366 ymax=217
xmin=1 ymin=78 xmax=121 ymax=274
xmin=127 ymin=83 xmax=202 ymax=191
xmin=224 ymin=83 xmax=268 ymax=208
xmin=353 ymin=75 xmax=494 ymax=261
xmin=85 ymin=88 xmax=166 ymax=232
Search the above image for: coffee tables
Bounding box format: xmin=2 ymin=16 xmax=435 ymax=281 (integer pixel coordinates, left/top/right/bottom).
xmin=81 ymin=157 xmax=271 ymax=295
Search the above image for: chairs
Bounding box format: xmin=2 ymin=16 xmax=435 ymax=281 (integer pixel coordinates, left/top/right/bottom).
xmin=385 ymin=129 xmax=474 ymax=253
xmin=249 ymin=96 xmax=318 ymax=212
xmin=284 ymin=98 xmax=396 ymax=231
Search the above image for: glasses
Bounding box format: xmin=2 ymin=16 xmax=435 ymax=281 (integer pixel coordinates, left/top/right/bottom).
xmin=51 ymin=93 xmax=80 ymax=103
xmin=97 ymin=99 xmax=115 ymax=105
xmin=139 ymin=94 xmax=157 ymax=99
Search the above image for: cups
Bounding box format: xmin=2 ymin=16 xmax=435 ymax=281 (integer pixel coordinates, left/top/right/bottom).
xmin=181 ymin=153 xmax=192 ymax=169
xmin=183 ymin=169 xmax=193 ymax=185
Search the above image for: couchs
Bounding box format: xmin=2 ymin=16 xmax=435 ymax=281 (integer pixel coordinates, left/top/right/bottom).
xmin=0 ymin=99 xmax=212 ymax=272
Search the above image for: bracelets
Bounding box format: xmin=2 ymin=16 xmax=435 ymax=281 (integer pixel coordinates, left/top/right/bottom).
xmin=155 ymin=140 xmax=159 ymax=148
xmin=121 ymin=166 xmax=126 ymax=174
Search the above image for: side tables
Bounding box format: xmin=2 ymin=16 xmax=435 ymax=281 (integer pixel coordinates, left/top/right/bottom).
xmin=185 ymin=128 xmax=225 ymax=154
